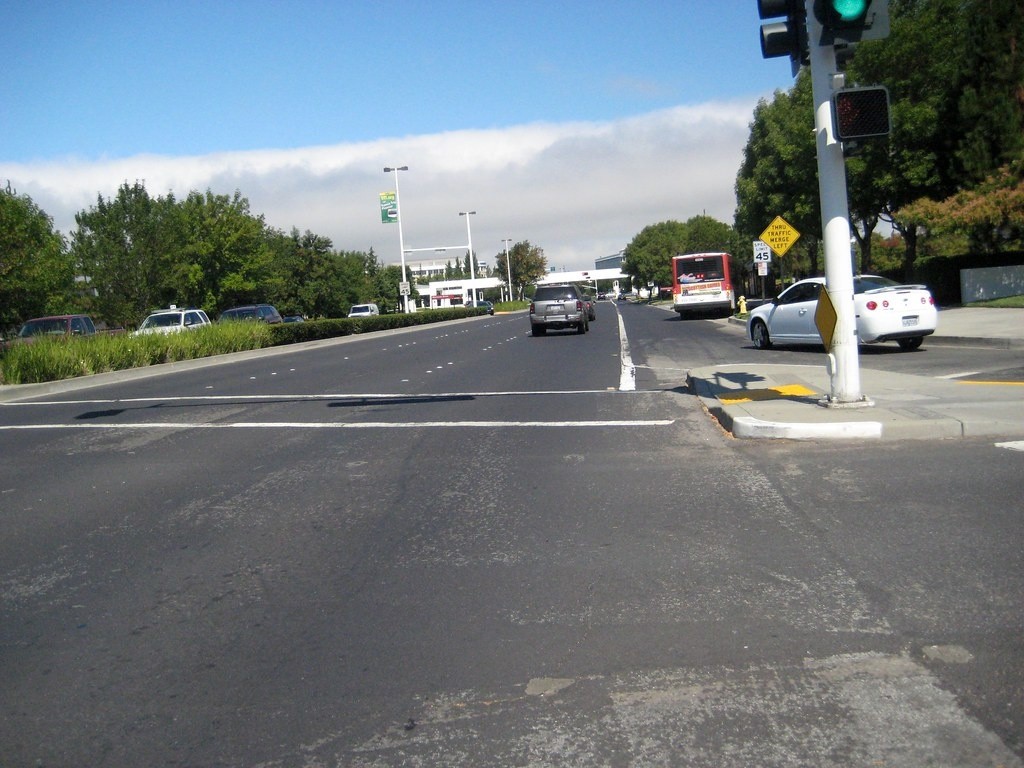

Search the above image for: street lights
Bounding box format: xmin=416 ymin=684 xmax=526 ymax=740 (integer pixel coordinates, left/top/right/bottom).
xmin=384 ymin=166 xmax=410 ymax=314
xmin=458 ymin=211 xmax=478 ymax=308
xmin=500 ymin=239 xmax=513 ymax=302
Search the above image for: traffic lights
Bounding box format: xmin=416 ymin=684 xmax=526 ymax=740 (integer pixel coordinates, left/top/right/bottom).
xmin=812 ymin=0 xmax=892 ymax=46
xmin=832 ymin=86 xmax=892 ymax=142
xmin=756 ymin=0 xmax=804 ymax=79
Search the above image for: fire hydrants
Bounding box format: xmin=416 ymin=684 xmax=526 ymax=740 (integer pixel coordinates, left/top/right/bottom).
xmin=738 ymin=296 xmax=748 ymax=314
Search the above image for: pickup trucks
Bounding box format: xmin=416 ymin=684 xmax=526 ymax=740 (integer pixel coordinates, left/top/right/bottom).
xmin=0 ymin=314 xmax=128 ymax=358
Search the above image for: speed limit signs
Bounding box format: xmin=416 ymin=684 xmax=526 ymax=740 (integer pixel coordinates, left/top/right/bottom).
xmin=752 ymin=241 xmax=773 ymax=264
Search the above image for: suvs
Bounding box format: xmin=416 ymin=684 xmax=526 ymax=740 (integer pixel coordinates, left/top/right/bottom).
xmin=215 ymin=304 xmax=283 ymax=326
xmin=348 ymin=303 xmax=379 ymax=318
xmin=598 ymin=292 xmax=606 ymax=301
xmin=529 ymin=285 xmax=591 ymax=338
xmin=129 ymin=305 xmax=212 ymax=338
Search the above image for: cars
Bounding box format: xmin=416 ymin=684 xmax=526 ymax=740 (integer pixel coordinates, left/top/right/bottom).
xmin=747 ymin=273 xmax=939 ymax=351
xmin=465 ymin=300 xmax=494 ymax=316
xmin=284 ymin=316 xmax=305 ymax=323
xmin=618 ymin=293 xmax=626 ymax=301
xmin=582 ymin=295 xmax=597 ymax=321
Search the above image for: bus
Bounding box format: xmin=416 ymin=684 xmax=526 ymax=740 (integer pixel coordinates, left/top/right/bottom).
xmin=672 ymin=252 xmax=739 ymax=320
